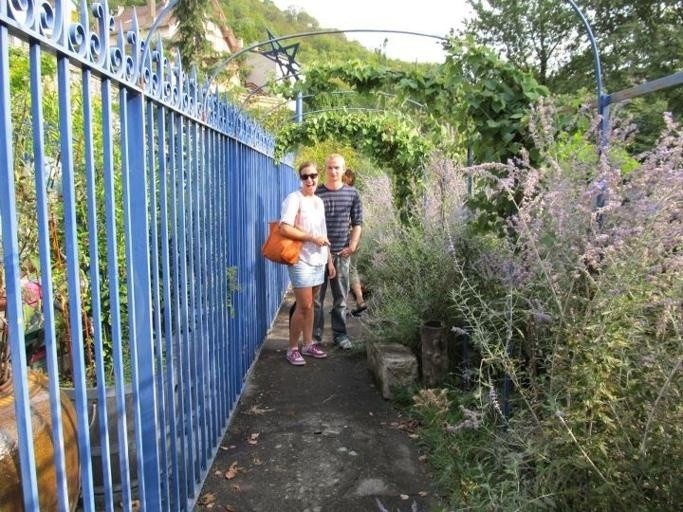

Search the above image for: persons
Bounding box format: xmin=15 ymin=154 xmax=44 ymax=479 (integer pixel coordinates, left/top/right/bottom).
xmin=339 ymin=168 xmax=368 ymax=317
xmin=312 ymin=153 xmax=363 ymax=353
xmin=277 ymin=159 xmax=338 ymax=368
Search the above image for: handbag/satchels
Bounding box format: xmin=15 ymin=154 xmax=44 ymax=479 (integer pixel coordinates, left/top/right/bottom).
xmin=262 ymin=220 xmax=306 ymax=266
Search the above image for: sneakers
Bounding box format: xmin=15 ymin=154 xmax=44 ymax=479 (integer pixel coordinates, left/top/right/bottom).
xmin=333 ymin=336 xmax=353 ymax=351
xmin=302 ymin=340 xmax=328 ymax=358
xmin=286 ymin=346 xmax=306 ymax=365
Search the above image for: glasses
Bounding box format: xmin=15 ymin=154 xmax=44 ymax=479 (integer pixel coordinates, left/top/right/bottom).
xmin=300 ymin=173 xmax=319 ymax=181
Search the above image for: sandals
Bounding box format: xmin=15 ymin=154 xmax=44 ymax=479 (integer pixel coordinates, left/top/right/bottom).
xmin=351 ymin=302 xmax=367 ymax=314
